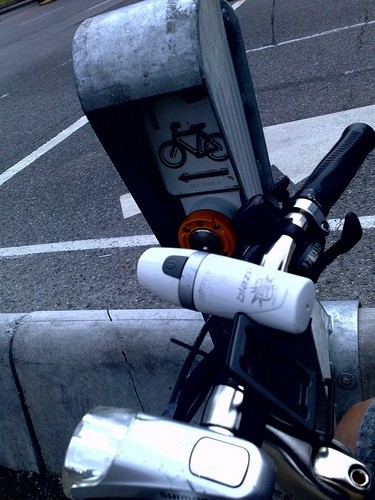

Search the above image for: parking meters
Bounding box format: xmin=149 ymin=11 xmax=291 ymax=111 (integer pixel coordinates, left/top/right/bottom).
xmin=73 ymin=0 xmax=273 ymax=258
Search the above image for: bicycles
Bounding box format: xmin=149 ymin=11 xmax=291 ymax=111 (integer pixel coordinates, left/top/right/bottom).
xmin=61 ymin=119 xmax=375 ymax=499
